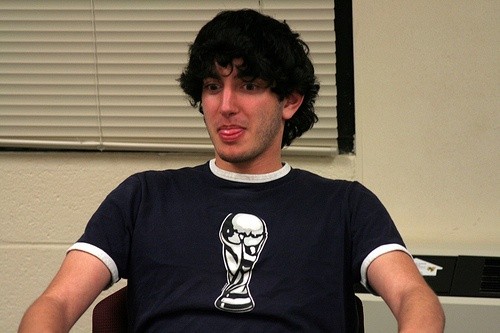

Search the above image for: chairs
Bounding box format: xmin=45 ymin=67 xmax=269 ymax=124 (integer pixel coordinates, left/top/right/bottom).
xmin=91 ymin=287 xmax=365 ymax=333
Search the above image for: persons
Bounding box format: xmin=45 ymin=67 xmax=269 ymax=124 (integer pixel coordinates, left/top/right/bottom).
xmin=17 ymin=8 xmax=446 ymax=333
